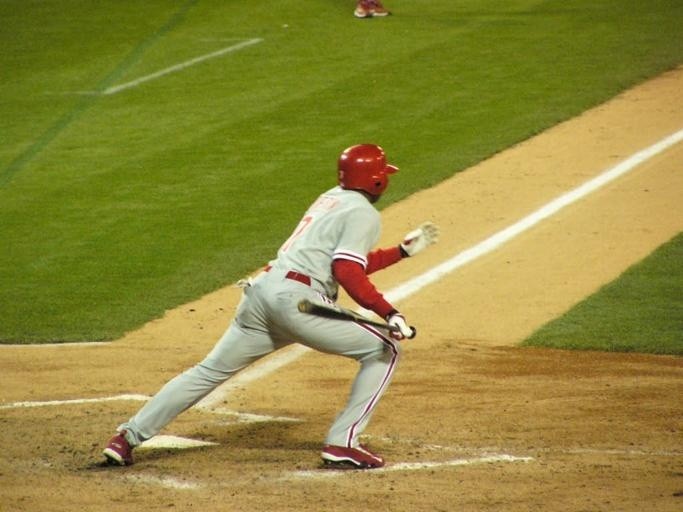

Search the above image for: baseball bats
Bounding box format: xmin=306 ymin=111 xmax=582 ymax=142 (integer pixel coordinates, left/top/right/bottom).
xmin=297 ymin=298 xmax=416 ymax=338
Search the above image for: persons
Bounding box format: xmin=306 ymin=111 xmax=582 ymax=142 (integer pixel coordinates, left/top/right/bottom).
xmin=100 ymin=142 xmax=441 ymax=469
xmin=351 ymin=1 xmax=391 ymax=19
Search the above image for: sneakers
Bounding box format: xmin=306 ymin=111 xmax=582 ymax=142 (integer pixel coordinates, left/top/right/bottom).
xmin=102 ymin=428 xmax=134 ymax=466
xmin=353 ymin=0 xmax=390 ymax=17
xmin=319 ymin=442 xmax=385 ymax=469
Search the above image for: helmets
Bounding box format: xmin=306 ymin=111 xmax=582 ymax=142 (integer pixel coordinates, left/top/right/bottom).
xmin=337 ymin=143 xmax=400 ymax=196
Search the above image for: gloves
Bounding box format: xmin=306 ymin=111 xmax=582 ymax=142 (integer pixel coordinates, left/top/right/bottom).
xmin=399 ymin=219 xmax=439 ymax=257
xmin=385 ymin=309 xmax=414 ymax=341
